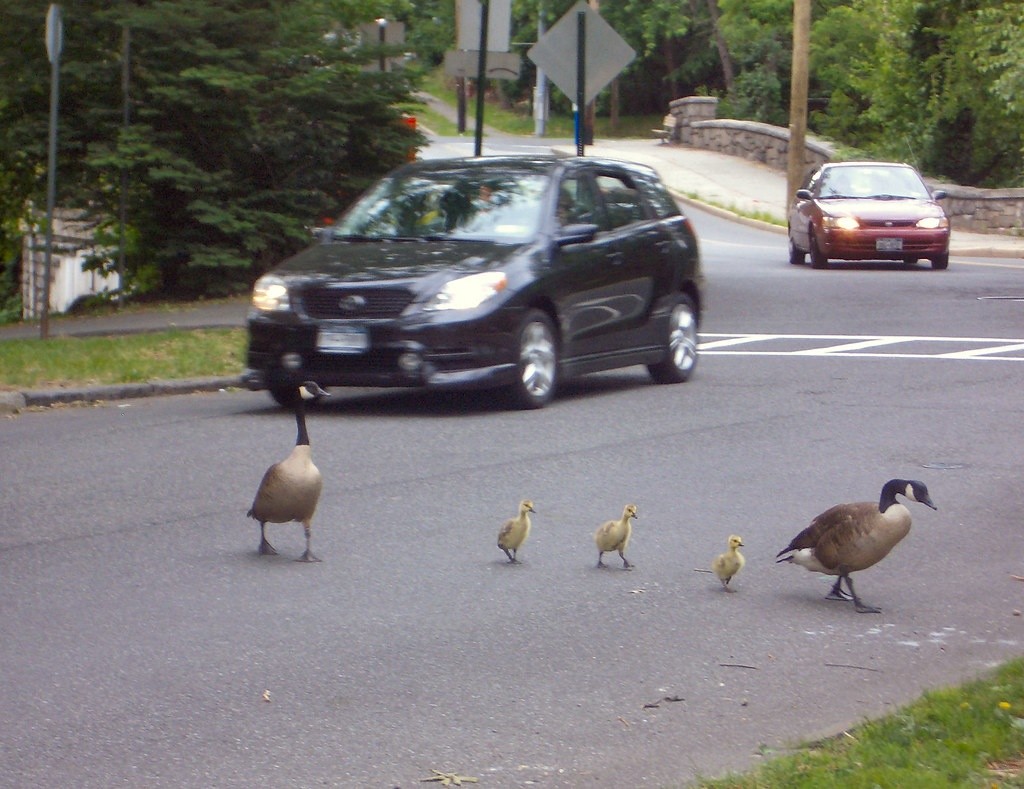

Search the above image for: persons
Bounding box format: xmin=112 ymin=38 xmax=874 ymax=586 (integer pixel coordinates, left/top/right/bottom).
xmin=539 ymin=186 xmax=580 ymax=228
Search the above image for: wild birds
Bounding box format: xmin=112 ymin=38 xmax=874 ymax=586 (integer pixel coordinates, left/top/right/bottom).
xmin=773 ymin=479 xmax=938 ymax=613
xmin=242 ymin=379 xmax=334 ymax=564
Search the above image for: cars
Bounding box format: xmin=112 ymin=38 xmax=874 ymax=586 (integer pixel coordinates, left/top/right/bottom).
xmin=239 ymin=155 xmax=704 ymax=412
xmin=787 ymin=161 xmax=951 ymax=270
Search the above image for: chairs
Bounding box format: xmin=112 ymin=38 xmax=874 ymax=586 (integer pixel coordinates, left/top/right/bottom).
xmin=438 ymin=190 xmax=477 ymax=233
xmin=602 ymin=186 xmax=642 ymax=227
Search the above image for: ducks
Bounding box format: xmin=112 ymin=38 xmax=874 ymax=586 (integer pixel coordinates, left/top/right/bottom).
xmin=498 ymin=498 xmax=537 ymax=565
xmin=593 ymin=505 xmax=639 ymax=570
xmin=711 ymin=534 xmax=745 ymax=592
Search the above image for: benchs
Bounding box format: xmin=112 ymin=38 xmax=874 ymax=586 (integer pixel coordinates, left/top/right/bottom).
xmin=650 ymin=113 xmax=677 ymax=144
